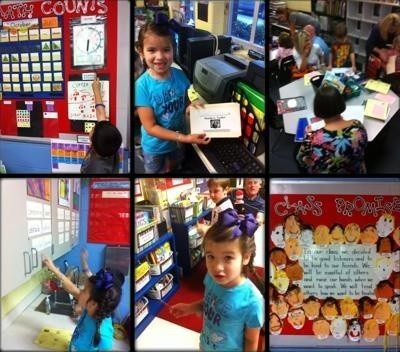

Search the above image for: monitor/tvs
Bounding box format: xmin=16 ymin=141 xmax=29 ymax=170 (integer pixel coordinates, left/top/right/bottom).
xmin=169 ymin=18 xmax=209 ymax=66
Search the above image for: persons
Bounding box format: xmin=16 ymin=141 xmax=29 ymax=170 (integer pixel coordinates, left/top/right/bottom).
xmin=196 ymin=178 xmax=233 ymax=240
xmin=79 ymin=77 xmax=122 ymax=173
xmin=42 ymin=256 xmax=122 ymax=352
xmin=134 ymin=20 xmax=212 ymax=176
xmin=80 ymin=246 xmax=125 ymax=288
xmin=169 ymin=208 xmax=265 ymax=351
xmin=271 ymin=7 xmax=399 ymax=96
xmin=228 ymin=178 xmax=265 ymax=214
xmin=295 ymin=85 xmax=370 ymax=176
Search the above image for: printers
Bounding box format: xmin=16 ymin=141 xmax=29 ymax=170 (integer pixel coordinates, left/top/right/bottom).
xmin=193 ymin=52 xmax=248 ymax=104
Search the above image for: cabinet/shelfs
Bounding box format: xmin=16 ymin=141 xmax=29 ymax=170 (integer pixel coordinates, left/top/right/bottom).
xmin=311 ymin=0 xmax=400 ymax=72
xmin=173 ymin=209 xmax=213 ymax=276
xmin=134 ymin=220 xmax=180 ymax=338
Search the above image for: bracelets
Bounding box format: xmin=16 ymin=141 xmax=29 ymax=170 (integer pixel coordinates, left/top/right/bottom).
xmin=82 ymin=268 xmax=91 ymax=273
xmin=57 ymin=273 xmax=65 ymax=287
xmin=175 ymin=130 xmax=184 ymax=150
xmin=95 ymin=104 xmax=105 ymax=108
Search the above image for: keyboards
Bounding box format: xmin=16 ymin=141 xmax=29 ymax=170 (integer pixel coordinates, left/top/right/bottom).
xmin=199 ymin=133 xmax=265 ymax=174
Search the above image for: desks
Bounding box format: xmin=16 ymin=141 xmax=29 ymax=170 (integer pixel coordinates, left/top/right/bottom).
xmin=278 ymin=68 xmax=400 ymax=144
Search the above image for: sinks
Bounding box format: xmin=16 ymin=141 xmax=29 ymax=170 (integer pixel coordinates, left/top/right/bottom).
xmin=49 ymin=288 xmax=75 ymax=304
xmin=34 ymin=301 xmax=73 ymax=317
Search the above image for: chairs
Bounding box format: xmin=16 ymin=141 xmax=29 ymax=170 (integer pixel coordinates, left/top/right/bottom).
xmin=267 ymin=97 xmax=283 ymax=152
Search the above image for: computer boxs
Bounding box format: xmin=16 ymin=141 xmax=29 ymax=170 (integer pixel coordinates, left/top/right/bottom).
xmin=242 ymin=58 xmax=265 ymax=157
xmin=186 ymin=34 xmax=232 ymax=84
